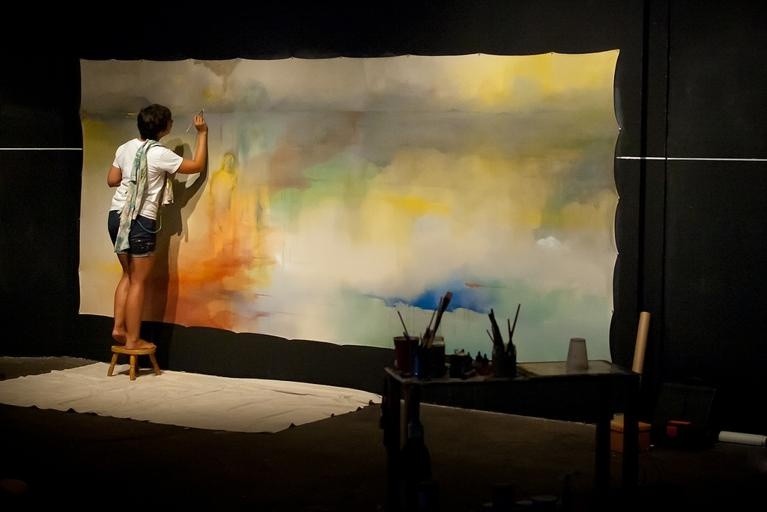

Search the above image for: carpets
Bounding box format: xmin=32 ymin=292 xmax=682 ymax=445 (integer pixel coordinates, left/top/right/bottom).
xmin=0 ymin=361 xmax=383 ymax=435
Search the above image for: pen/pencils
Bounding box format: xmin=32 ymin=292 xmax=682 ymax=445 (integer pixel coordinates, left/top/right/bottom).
xmin=186 ymin=109 xmax=202 ymax=135
xmin=486 ymin=303 xmax=521 ymax=354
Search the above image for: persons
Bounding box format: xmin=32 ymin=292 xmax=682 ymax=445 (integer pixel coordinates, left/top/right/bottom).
xmin=104 ymin=104 xmax=207 ymax=350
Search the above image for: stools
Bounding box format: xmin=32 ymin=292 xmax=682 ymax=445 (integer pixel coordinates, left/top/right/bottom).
xmin=106 ymin=346 xmax=161 ymax=381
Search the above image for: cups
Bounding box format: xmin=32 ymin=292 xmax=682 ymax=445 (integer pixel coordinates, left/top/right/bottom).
xmin=492 ymin=343 xmax=519 ymax=379
xmin=394 ymin=336 xmax=420 ymax=376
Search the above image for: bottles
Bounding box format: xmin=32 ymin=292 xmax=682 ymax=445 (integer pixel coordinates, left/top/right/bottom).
xmin=430 ymin=336 xmax=446 ymax=378
xmin=566 ymin=338 xmax=588 ymax=370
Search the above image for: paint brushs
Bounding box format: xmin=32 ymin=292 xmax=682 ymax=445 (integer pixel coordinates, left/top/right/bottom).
xmin=397 ymin=291 xmax=453 ymax=348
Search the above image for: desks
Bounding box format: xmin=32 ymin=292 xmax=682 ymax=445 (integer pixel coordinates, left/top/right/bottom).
xmin=376 ymin=360 xmax=640 ymax=512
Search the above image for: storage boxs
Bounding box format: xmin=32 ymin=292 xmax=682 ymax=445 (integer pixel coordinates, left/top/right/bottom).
xmin=609 ymin=421 xmax=651 ymax=455
xmin=653 ymin=417 xmax=714 ymax=453
